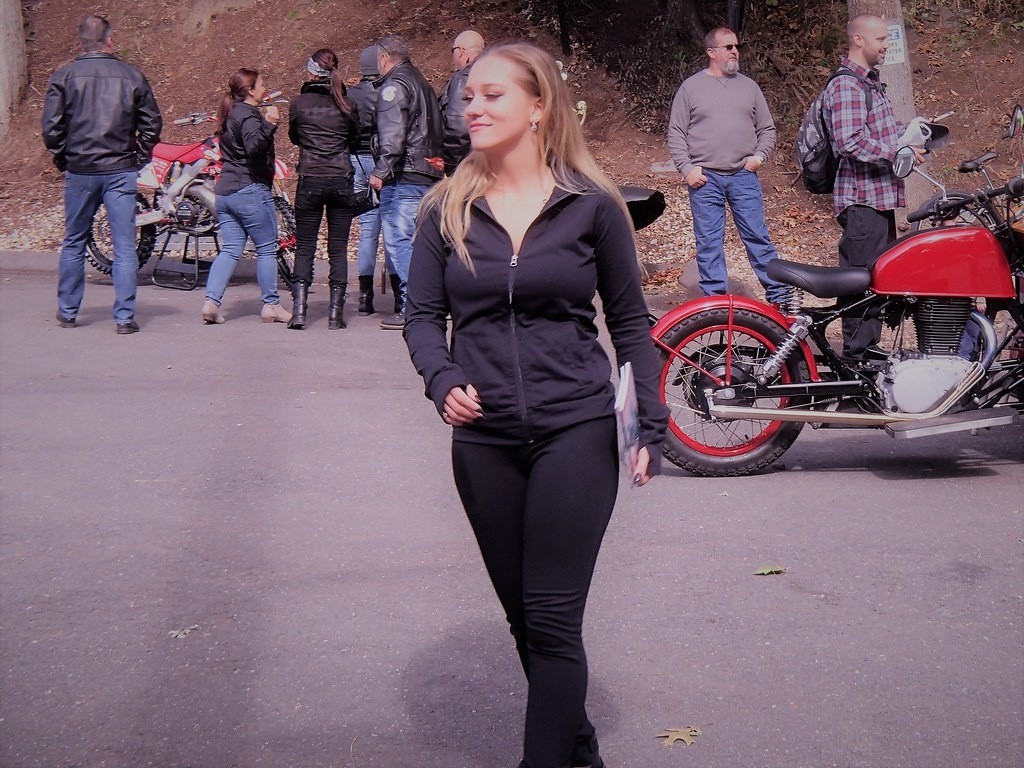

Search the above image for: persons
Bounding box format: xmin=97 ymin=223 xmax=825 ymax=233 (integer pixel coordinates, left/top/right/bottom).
xmin=369 ymin=41 xmax=445 ymax=329
xmin=346 ymin=46 xmax=403 ymax=316
xmin=667 ymin=28 xmax=791 ymax=305
xmin=202 ymin=68 xmax=292 ymax=323
xmin=436 ymin=30 xmax=484 ymax=176
xmin=822 ymin=13 xmax=933 ymax=358
xmin=41 ymin=16 xmax=163 ymax=333
xmin=403 ymin=42 xmax=672 ymax=768
xmin=286 ymin=49 xmax=362 ymax=330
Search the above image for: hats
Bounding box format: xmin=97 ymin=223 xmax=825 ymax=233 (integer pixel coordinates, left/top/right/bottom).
xmin=360 ymin=45 xmax=381 ymax=75
xmin=918 ymin=120 xmax=949 ymax=153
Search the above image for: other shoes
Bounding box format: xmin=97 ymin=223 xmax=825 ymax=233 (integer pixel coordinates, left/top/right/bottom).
xmin=776 ymin=305 xmax=808 ymax=318
xmin=202 ymin=301 xmax=225 ymax=324
xmin=260 ymin=303 xmax=292 ymax=323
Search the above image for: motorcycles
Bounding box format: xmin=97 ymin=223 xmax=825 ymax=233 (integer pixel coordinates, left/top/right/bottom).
xmin=83 ymin=91 xmax=297 ymax=289
xmin=650 ymin=103 xmax=1024 ymax=477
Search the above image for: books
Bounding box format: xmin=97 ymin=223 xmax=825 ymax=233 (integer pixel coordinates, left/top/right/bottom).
xmin=614 ymin=361 xmax=639 ymax=490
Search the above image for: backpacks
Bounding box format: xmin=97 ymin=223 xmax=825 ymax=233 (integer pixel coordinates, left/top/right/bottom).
xmin=795 ymin=70 xmax=871 ymax=195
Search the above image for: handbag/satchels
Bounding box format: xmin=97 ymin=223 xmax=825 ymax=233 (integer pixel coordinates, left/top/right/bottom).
xmin=352 ymin=188 xmax=378 ymax=218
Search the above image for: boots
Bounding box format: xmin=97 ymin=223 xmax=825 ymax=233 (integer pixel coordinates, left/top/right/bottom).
xmin=329 ymin=285 xmax=350 ymax=330
xmin=358 ymin=274 xmax=375 ymax=316
xmin=287 ymin=280 xmax=308 ymax=328
xmin=389 ymin=274 xmax=403 ymax=314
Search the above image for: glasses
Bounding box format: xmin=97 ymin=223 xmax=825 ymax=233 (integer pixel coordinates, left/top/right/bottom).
xmin=451 ymin=46 xmax=465 ymax=54
xmin=710 ymin=44 xmax=741 ymax=51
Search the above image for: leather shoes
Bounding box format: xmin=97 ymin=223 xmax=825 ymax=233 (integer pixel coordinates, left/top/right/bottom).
xmin=864 ymin=345 xmax=891 ymax=360
xmin=381 ymin=312 xmax=405 ymax=329
xmin=117 ymin=321 xmax=139 ymax=334
xmin=56 ymin=309 xmax=76 ymax=328
